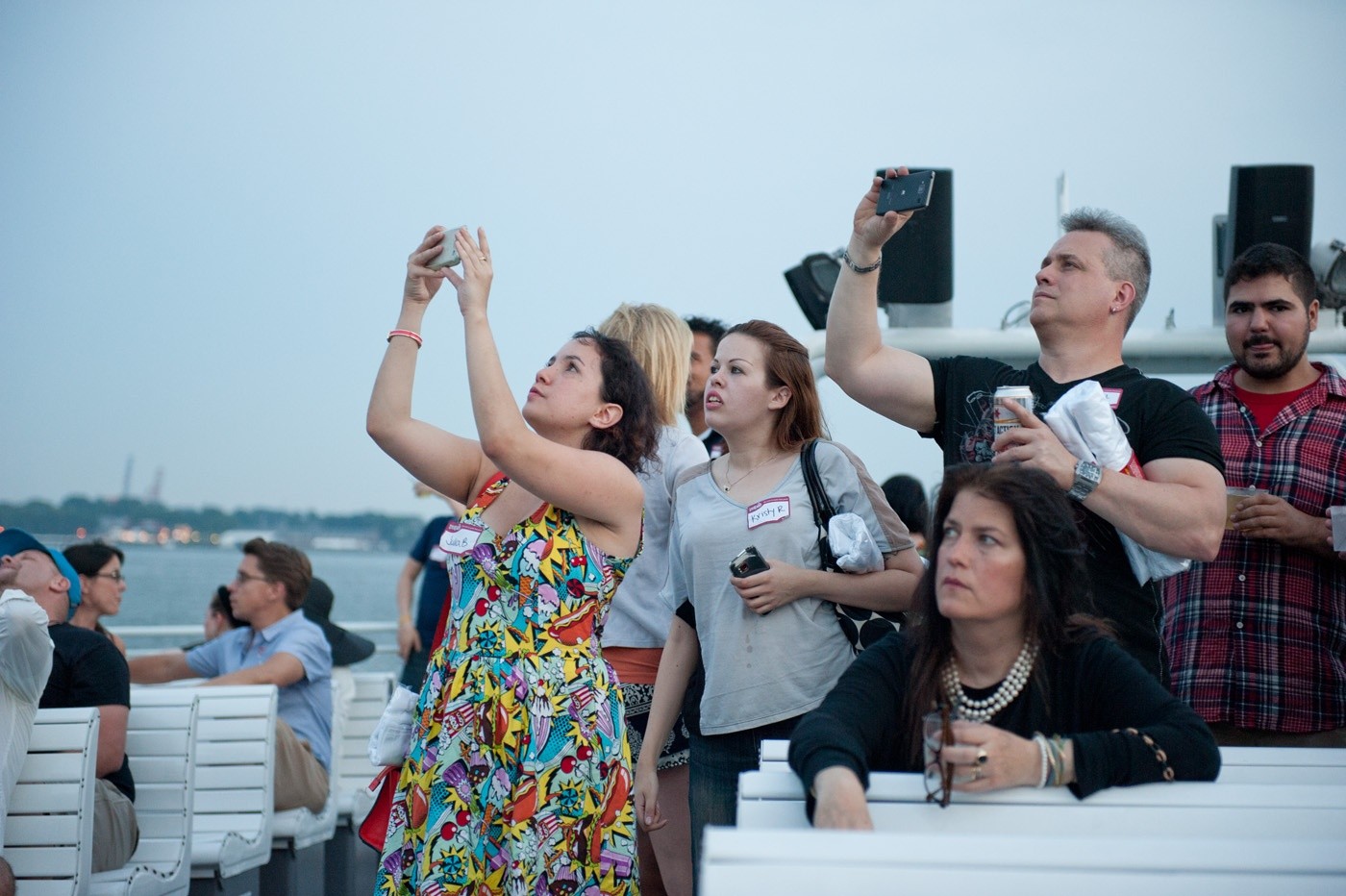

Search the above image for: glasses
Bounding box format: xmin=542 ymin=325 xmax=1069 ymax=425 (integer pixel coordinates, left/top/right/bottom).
xmin=919 ymin=704 xmax=956 ymax=807
xmin=238 ymin=569 xmax=268 ymax=584
xmin=96 ymin=570 xmax=125 ymax=581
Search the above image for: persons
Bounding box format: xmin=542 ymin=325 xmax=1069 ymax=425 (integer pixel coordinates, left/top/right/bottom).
xmin=364 ymin=226 xmax=664 ymax=896
xmin=60 ymin=538 xmax=129 ymax=660
xmin=1 ymin=526 xmax=139 ymax=875
xmin=631 ymin=318 xmax=924 ymax=896
xmin=1324 ymin=503 xmax=1346 ymax=557
xmin=784 ymin=464 xmax=1225 ymax=835
xmin=125 ymin=533 xmax=336 ymax=816
xmin=165 ymin=585 xmax=250 ymax=652
xmin=1157 ymin=244 xmax=1346 ymax=749
xmin=681 ymin=314 xmax=729 ymax=463
xmin=301 ymin=577 xmax=377 ymax=789
xmin=593 ymin=300 xmax=718 ymax=892
xmin=822 ymin=166 xmax=1230 ymax=700
xmin=0 ymin=581 xmax=56 ymax=896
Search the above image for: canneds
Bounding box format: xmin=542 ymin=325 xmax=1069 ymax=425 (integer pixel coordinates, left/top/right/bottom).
xmin=989 ymin=384 xmax=1034 ymax=464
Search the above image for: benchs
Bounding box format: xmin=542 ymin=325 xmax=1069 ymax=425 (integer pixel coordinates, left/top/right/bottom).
xmin=0 ymin=658 xmax=392 ymax=896
xmin=694 ymin=733 xmax=1342 ymax=896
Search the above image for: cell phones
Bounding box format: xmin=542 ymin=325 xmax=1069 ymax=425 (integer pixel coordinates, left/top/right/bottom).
xmin=876 ymin=169 xmax=936 ymax=215
xmin=425 ymin=225 xmax=467 ymax=269
xmin=728 ymin=545 xmax=773 ymax=616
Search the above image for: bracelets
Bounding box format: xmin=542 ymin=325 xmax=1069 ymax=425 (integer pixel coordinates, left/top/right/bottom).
xmin=383 ymin=330 xmax=423 ymax=348
xmin=842 ymin=250 xmax=882 ymax=274
xmin=1031 ymin=730 xmax=1067 ymax=789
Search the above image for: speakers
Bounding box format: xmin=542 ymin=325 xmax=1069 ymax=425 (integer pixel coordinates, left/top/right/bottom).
xmin=878 ymin=166 xmax=952 ymax=305
xmin=1226 ymin=163 xmax=1315 ymax=273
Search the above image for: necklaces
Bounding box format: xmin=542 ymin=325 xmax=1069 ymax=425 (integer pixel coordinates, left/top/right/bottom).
xmin=936 ymin=634 xmax=1039 ymax=725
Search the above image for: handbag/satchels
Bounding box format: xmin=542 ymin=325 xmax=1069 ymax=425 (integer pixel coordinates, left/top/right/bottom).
xmin=359 ymin=764 xmax=402 ymax=855
xmin=818 ymin=509 xmax=910 ymax=655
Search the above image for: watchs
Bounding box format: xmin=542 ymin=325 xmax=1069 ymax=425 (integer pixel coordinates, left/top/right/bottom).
xmin=1067 ymin=460 xmax=1103 ymax=503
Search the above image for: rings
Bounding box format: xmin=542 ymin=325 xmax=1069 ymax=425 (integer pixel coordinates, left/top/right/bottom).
xmin=976 ymin=746 xmax=989 ymax=767
xmin=970 ymin=766 xmax=983 ymax=780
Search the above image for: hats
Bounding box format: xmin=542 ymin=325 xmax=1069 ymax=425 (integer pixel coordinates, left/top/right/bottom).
xmin=299 ymin=576 xmax=376 ymax=668
xmin=0 ymin=527 xmax=81 ymax=625
